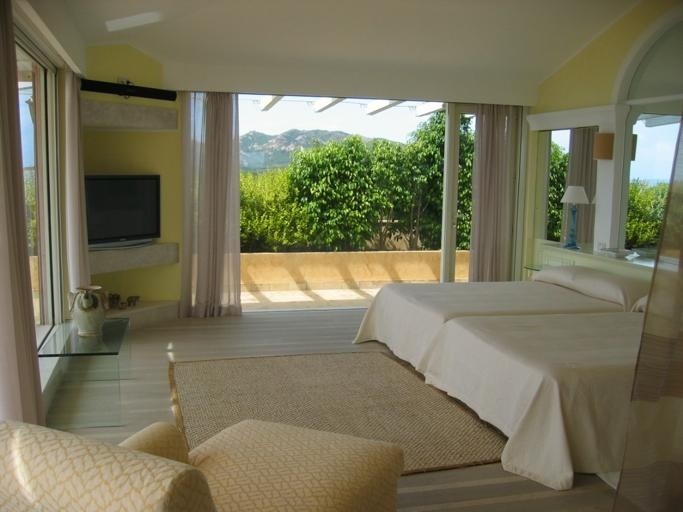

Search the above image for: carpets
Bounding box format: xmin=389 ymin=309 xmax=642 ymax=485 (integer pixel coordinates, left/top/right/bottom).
xmin=166 ymin=346 xmax=510 ymax=477
xmin=592 ymin=453 xmax=682 ymax=510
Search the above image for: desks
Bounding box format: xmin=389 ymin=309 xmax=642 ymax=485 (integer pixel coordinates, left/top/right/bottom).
xmin=38 ymin=315 xmax=134 ymax=431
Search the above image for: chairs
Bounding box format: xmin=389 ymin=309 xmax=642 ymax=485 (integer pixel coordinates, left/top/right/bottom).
xmin=1 ymin=407 xmax=407 ymax=512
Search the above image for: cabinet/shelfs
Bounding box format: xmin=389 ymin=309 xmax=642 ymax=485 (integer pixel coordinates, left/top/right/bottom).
xmin=86 ymin=241 xmax=181 ymax=328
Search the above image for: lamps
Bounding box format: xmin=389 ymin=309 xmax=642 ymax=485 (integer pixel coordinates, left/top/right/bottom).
xmin=629 ymin=132 xmax=638 ymax=162
xmin=591 ymin=130 xmax=614 ymax=160
xmin=559 ymin=184 xmax=591 ymax=249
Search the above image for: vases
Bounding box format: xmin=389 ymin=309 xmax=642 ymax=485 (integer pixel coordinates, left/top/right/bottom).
xmin=71 ymin=285 xmax=105 ymax=338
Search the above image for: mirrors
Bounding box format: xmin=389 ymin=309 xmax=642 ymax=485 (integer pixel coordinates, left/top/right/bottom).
xmin=619 ymin=106 xmax=681 ymax=262
xmin=532 ymin=120 xmax=600 ymax=256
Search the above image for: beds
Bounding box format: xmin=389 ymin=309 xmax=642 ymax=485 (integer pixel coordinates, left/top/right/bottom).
xmin=350 ymin=262 xmax=653 ymax=377
xmin=423 ymin=288 xmax=681 ymax=492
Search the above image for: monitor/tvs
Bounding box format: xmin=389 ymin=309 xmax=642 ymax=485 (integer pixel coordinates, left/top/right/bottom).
xmin=84 ymin=174 xmax=164 ymax=249
